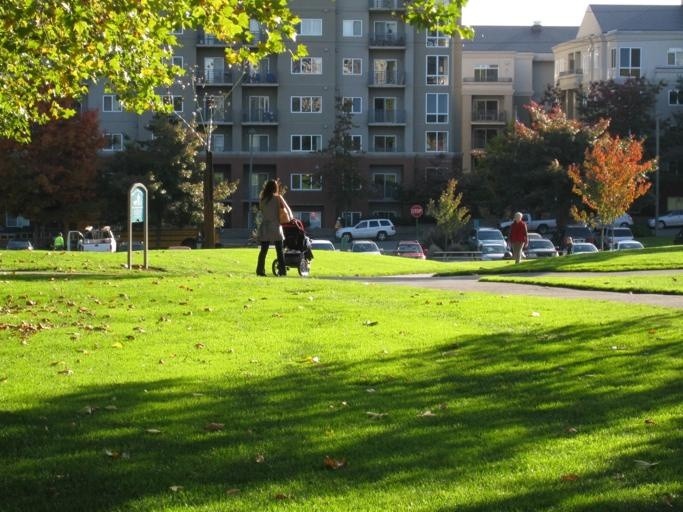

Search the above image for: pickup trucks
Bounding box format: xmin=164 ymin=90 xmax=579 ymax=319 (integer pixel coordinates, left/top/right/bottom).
xmin=67 ymin=226 xmax=117 ymax=254
xmin=500 ymin=213 xmax=557 ymax=235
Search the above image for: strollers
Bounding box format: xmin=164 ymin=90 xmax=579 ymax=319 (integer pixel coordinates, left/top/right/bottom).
xmin=272 ymin=218 xmax=314 ymax=277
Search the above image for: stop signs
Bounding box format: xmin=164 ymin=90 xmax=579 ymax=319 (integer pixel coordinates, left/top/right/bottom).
xmin=410 ymin=204 xmax=424 ymax=218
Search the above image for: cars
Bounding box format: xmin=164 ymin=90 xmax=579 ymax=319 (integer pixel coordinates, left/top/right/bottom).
xmin=6 ymin=238 xmax=33 ymax=250
xmin=567 ymin=212 xmax=645 ymax=256
xmin=472 ymin=227 xmax=560 ymax=261
xmin=336 ymin=218 xmax=397 ymax=242
xmin=310 ymin=239 xmax=340 ymax=252
xmin=348 ymin=239 xmax=384 ymax=256
xmin=648 ymin=209 xmax=683 ymax=230
xmin=393 ymin=240 xmax=428 ymax=260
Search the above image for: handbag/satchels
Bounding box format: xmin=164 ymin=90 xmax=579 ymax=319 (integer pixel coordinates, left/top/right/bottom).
xmin=566 ymin=240 xmax=573 ymax=245
xmin=278 ymin=205 xmax=290 ymax=225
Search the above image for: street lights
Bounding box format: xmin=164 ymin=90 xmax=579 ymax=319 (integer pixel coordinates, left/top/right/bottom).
xmin=247 ymin=128 xmax=256 ymax=235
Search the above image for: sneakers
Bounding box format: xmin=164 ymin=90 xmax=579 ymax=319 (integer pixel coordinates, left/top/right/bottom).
xmin=257 ymin=272 xmax=266 ymax=276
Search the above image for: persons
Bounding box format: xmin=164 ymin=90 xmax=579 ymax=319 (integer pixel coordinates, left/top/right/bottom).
xmin=54 ymin=232 xmax=64 ymax=251
xmin=335 ymin=217 xmax=342 ymax=230
xmin=255 ymin=179 xmax=293 ymax=276
xmin=508 ymin=212 xmax=528 ymax=264
xmin=566 ymin=236 xmax=573 ymax=255
